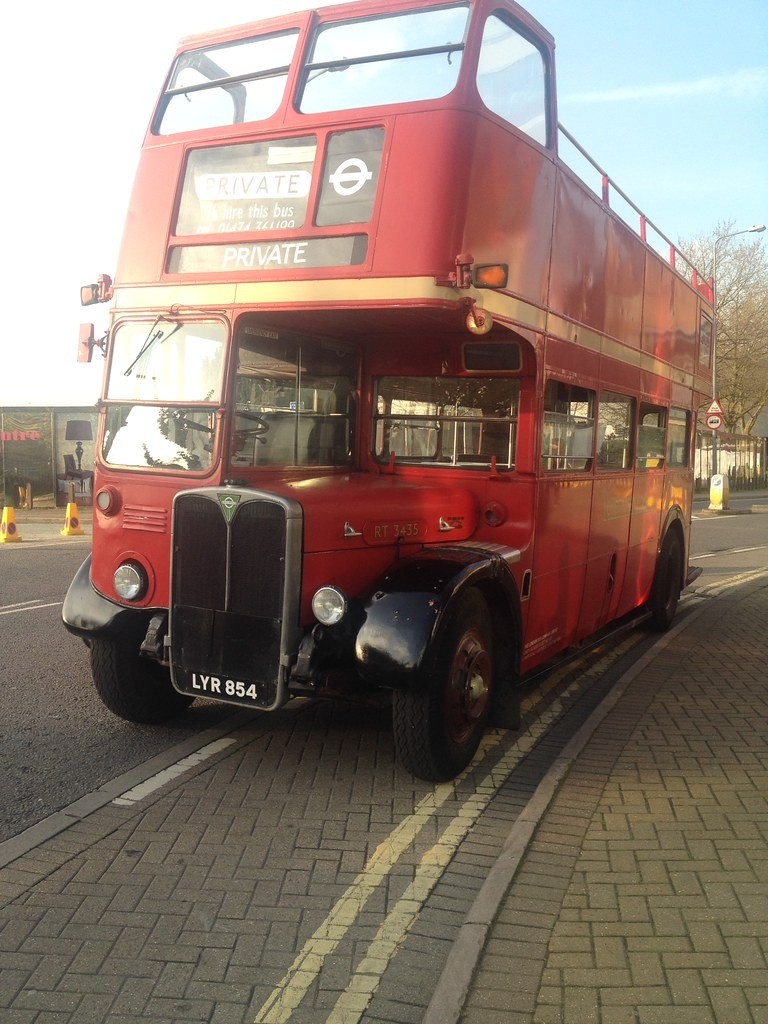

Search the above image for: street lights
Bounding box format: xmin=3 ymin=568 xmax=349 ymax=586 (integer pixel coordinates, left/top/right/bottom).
xmin=709 ymin=223 xmax=767 ymax=475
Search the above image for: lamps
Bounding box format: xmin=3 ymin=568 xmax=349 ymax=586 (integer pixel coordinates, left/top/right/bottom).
xmin=65 ymin=420 xmax=93 ymax=470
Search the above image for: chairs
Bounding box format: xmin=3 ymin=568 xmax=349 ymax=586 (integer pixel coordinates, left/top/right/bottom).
xmin=250 ymin=411 xmax=348 ymax=469
xmin=64 ymin=455 xmax=95 ymax=493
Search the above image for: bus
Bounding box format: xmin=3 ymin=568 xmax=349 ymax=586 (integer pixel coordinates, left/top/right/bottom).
xmin=60 ymin=2 xmax=707 ymax=783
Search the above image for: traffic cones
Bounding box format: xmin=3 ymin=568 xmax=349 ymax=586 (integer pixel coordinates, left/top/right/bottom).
xmin=0 ymin=506 xmax=23 ymax=544
xmin=60 ymin=502 xmax=85 ymax=536
